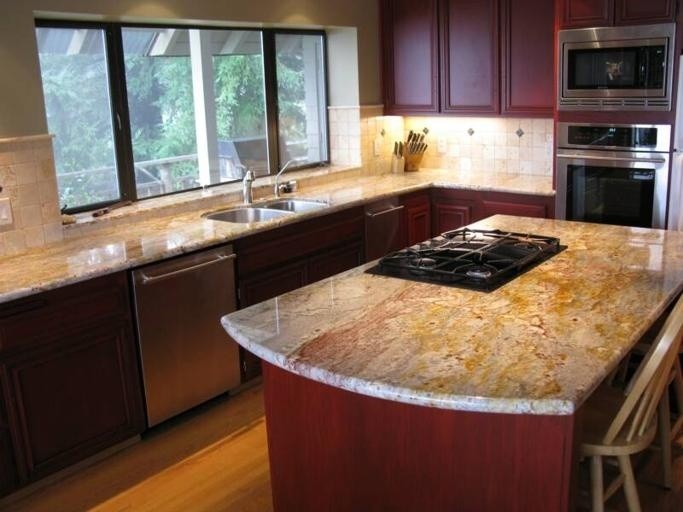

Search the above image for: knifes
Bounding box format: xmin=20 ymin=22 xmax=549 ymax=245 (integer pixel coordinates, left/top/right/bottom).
xmin=393 ymin=128 xmax=428 ymax=160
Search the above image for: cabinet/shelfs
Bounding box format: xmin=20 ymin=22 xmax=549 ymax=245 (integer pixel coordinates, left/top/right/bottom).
xmin=437 ymin=1 xmax=557 ymax=118
xmin=398 ymin=188 xmax=433 ymax=248
xmin=379 ymin=0 xmax=440 ymax=115
xmin=431 ymin=186 xmax=556 ymax=239
xmin=1 ymin=269 xmax=144 ymax=499
xmin=557 ymin=0 xmax=676 ymax=30
xmin=230 ymin=204 xmax=364 ymax=388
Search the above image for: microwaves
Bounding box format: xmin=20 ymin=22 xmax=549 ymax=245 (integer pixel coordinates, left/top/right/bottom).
xmin=554 ymin=23 xmax=673 ymax=115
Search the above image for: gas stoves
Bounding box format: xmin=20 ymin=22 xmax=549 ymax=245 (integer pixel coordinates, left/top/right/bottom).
xmin=361 ymin=224 xmax=568 ymax=296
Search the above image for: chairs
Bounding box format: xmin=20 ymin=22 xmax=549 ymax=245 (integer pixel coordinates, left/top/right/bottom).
xmin=620 ymin=338 xmax=683 ymax=490
xmin=575 ymin=294 xmax=683 ymax=512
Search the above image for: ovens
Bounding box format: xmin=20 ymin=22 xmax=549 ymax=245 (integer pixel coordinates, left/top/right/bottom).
xmin=551 ymin=121 xmax=675 ymax=232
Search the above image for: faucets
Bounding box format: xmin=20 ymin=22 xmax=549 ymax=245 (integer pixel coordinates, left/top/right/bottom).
xmin=243 ymin=169 xmax=257 ymax=203
xmin=274 ymin=159 xmax=299 ymax=197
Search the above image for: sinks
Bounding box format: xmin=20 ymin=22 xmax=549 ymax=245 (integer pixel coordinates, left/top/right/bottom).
xmin=201 ymin=205 xmax=295 ymax=222
xmin=259 ymin=197 xmax=334 ymax=213
